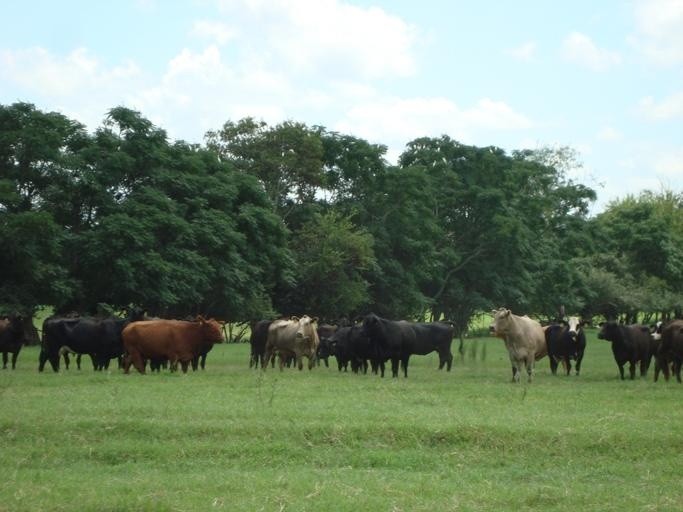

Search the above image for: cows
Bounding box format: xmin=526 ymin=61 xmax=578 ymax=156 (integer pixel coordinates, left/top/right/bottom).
xmin=0 ymin=311 xmax=24 ymax=370
xmin=36 ymin=308 xmax=147 ymax=375
xmin=248 ymin=313 xmax=458 ymax=382
xmin=122 ymin=315 xmax=226 ymax=377
xmin=596 ymin=317 xmax=653 ymax=381
xmin=489 ymin=306 xmax=548 ymax=386
xmin=651 ymin=318 xmax=683 ymax=384
xmin=544 ymin=315 xmax=587 ymax=378
